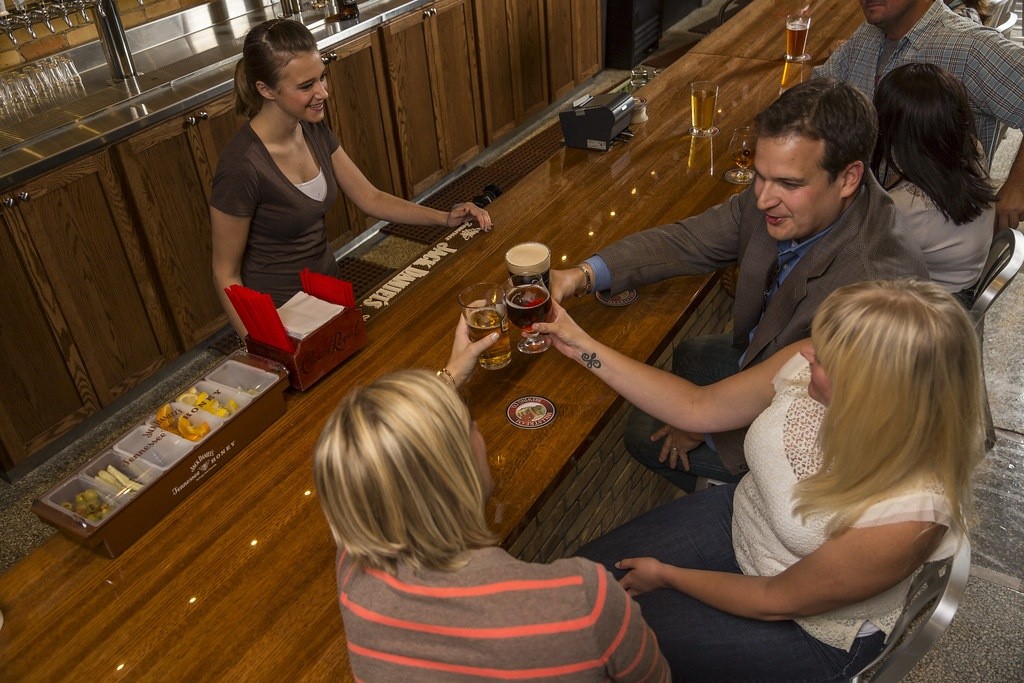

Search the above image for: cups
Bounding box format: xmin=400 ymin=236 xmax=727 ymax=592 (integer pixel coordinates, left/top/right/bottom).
xmin=779 ymin=60 xmax=803 ymax=94
xmin=0 ymin=56 xmax=82 ymax=114
xmin=456 ymin=282 xmax=511 ymax=370
xmin=690 ymin=81 xmax=719 ymax=133
xmin=1 ymin=76 xmax=86 ymax=124
xmin=786 ymin=13 xmax=811 ymax=60
xmin=686 ymin=133 xmax=713 ymax=176
xmin=630 ymin=68 xmax=666 ymax=87
xmin=505 ymin=241 xmax=551 ymax=290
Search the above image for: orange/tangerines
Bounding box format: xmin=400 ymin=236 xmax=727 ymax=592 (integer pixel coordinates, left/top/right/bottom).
xmin=156 ymin=403 xmax=211 ymax=443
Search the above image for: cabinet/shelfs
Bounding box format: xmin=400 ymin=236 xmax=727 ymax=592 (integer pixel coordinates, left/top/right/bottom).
xmin=1 ymin=1 xmax=605 ymax=467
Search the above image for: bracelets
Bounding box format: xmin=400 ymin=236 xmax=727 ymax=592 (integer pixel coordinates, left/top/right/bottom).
xmin=571 ymin=264 xmax=591 ymax=299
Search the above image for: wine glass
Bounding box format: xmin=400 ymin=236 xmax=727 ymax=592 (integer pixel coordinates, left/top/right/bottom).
xmin=503 ymin=271 xmax=552 ymax=353
xmin=728 ymin=126 xmax=755 ymax=182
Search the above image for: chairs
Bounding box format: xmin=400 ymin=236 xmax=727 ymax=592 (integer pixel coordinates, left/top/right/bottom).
xmin=695 ymin=9 xmax=1024 ymax=682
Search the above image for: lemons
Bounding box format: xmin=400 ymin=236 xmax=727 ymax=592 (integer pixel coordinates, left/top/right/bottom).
xmin=177 ymin=387 xmax=240 ymax=417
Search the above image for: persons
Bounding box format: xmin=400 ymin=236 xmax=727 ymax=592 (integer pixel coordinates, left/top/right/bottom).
xmin=875 ymin=62 xmax=1001 ymax=308
xmin=209 ymin=18 xmax=490 ymax=348
xmin=311 ymin=364 xmax=675 ymax=683
xmin=809 ymin=0 xmax=1024 ymax=191
xmin=546 ymin=75 xmax=934 ymax=493
xmin=537 ymin=284 xmax=983 ymax=683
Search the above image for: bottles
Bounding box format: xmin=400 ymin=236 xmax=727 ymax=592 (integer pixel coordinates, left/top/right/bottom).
xmin=334 ymin=0 xmax=359 ymax=21
xmin=632 ymin=96 xmax=648 ymax=123
